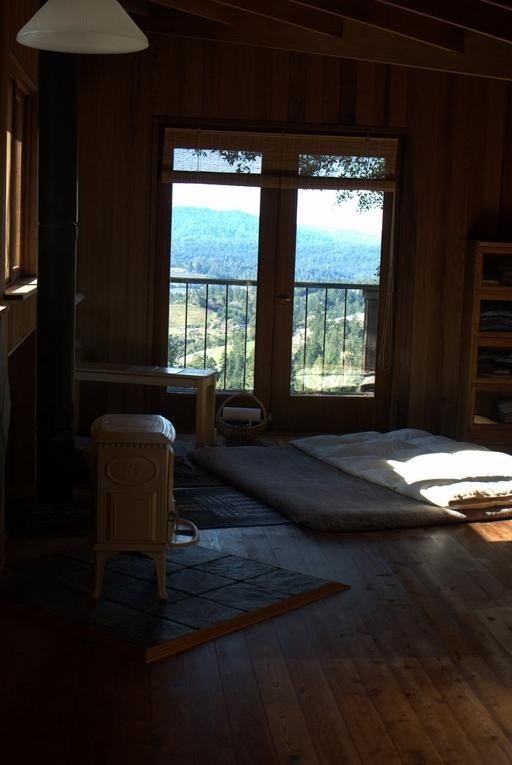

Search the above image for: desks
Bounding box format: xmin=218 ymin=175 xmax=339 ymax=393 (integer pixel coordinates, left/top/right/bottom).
xmin=70 ymin=362 xmax=218 ymax=449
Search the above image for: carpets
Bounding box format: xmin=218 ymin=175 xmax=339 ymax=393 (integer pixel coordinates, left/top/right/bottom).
xmin=6 ymin=487 xmax=294 ymax=536
xmin=172 ymin=453 xmax=229 ymax=487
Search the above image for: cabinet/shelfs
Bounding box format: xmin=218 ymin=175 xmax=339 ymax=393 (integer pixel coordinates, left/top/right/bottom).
xmin=90 ymin=412 xmax=201 ymax=602
xmin=453 ymin=237 xmax=512 ymax=452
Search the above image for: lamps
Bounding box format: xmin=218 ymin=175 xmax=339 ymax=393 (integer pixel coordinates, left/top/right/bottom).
xmin=17 ymin=0 xmax=150 ymax=56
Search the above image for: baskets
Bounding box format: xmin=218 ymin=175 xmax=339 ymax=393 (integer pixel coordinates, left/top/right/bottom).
xmin=215 ymin=391 xmax=267 ymax=440
